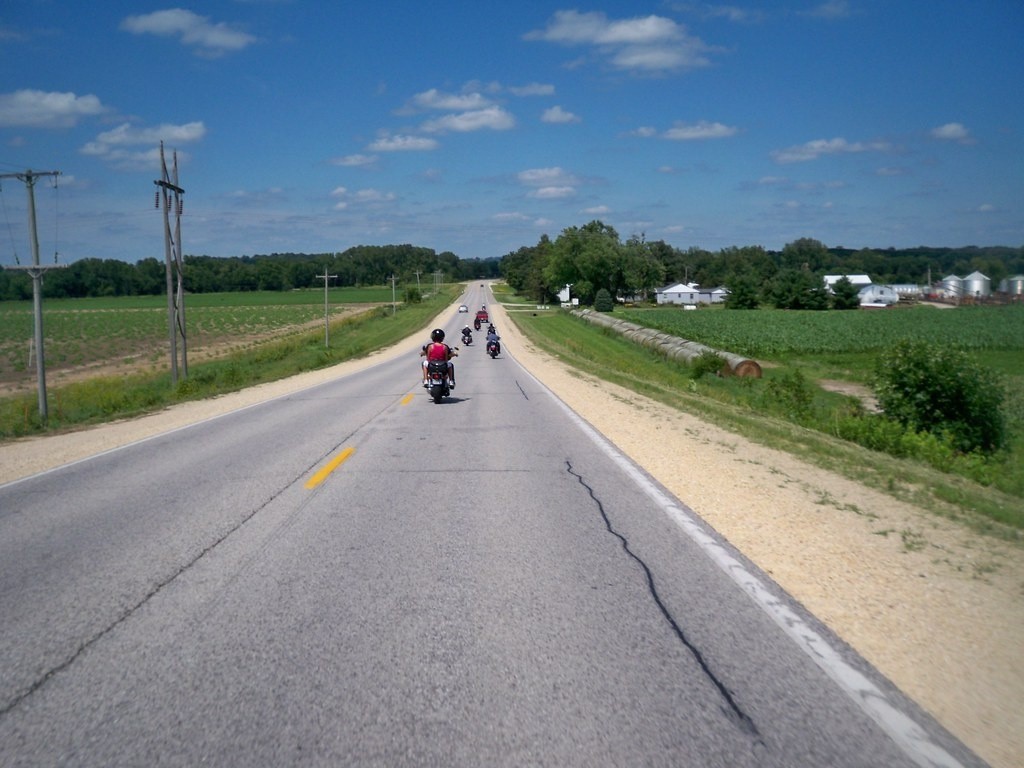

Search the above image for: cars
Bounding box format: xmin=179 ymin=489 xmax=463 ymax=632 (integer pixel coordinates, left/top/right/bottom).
xmin=459 ymin=305 xmax=468 ymax=313
xmin=476 ymin=310 xmax=489 ymax=323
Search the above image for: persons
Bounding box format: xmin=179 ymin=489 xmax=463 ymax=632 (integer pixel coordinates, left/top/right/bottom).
xmin=419 ymin=328 xmax=459 ymax=390
xmin=474 ymin=316 xmax=481 ymax=328
xmin=488 ymin=322 xmax=495 ymax=334
xmin=462 ymin=324 xmax=472 ymax=343
xmin=485 ymin=329 xmax=501 ymax=353
xmin=482 ymin=303 xmax=485 ymax=310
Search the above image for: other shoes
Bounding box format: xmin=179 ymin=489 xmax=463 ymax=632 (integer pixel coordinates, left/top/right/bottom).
xmin=422 ymin=379 xmax=429 ymax=388
xmin=487 ymin=352 xmax=489 ymax=354
xmin=498 ymin=352 xmax=500 ymax=353
xmin=449 ymin=380 xmax=455 ymax=389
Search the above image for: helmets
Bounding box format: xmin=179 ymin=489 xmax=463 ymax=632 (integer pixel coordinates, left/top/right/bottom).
xmin=466 ymin=325 xmax=468 ymax=327
xmin=431 ymin=329 xmax=445 ymax=342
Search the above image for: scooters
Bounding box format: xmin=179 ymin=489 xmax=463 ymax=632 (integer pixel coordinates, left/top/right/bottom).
xmin=486 ymin=339 xmax=498 ymax=359
xmin=475 ymin=323 xmax=481 ymax=331
xmin=420 ymin=345 xmax=459 ymax=404
xmin=463 ymin=332 xmax=471 ymax=346
